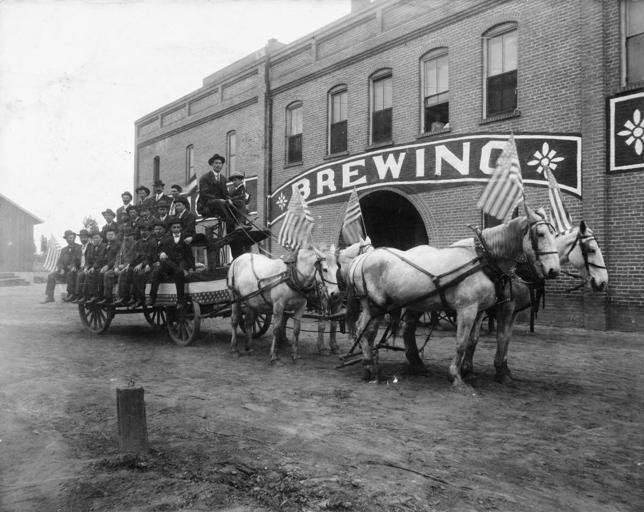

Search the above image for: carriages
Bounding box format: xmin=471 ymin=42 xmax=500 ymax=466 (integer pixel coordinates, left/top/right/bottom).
xmin=79 ymin=203 xmax=608 ymax=397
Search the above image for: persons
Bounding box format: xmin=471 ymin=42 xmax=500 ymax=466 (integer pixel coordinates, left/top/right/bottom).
xmin=40 ymin=153 xmax=252 ymax=310
xmin=431 ymin=112 xmax=449 ymax=130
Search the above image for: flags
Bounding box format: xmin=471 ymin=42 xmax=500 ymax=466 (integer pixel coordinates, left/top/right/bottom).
xmin=342 ymin=189 xmax=363 ymax=246
xmin=476 ymin=134 xmax=524 ymax=223
xmin=547 ymin=169 xmax=573 ymax=234
xmin=277 ymin=187 xmax=314 ymax=252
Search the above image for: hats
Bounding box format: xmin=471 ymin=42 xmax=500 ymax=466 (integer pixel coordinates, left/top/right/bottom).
xmin=62 ymin=179 xmax=189 ymax=239
xmin=208 ymin=153 xmax=226 ymax=165
xmin=228 ymin=171 xmax=244 ymax=179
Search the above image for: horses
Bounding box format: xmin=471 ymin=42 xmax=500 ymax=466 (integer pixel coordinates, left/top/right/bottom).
xmin=391 ymin=219 xmax=609 ymax=387
xmin=336 ymin=202 xmax=561 ymax=393
xmin=226 ymin=243 xmax=341 ymax=367
xmin=277 ymin=233 xmax=375 ymax=349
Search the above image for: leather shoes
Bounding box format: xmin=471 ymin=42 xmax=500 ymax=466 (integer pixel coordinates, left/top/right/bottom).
xmin=41 ymin=295 xmax=185 ymax=309
xmin=234 ymin=222 xmax=253 ymax=232
